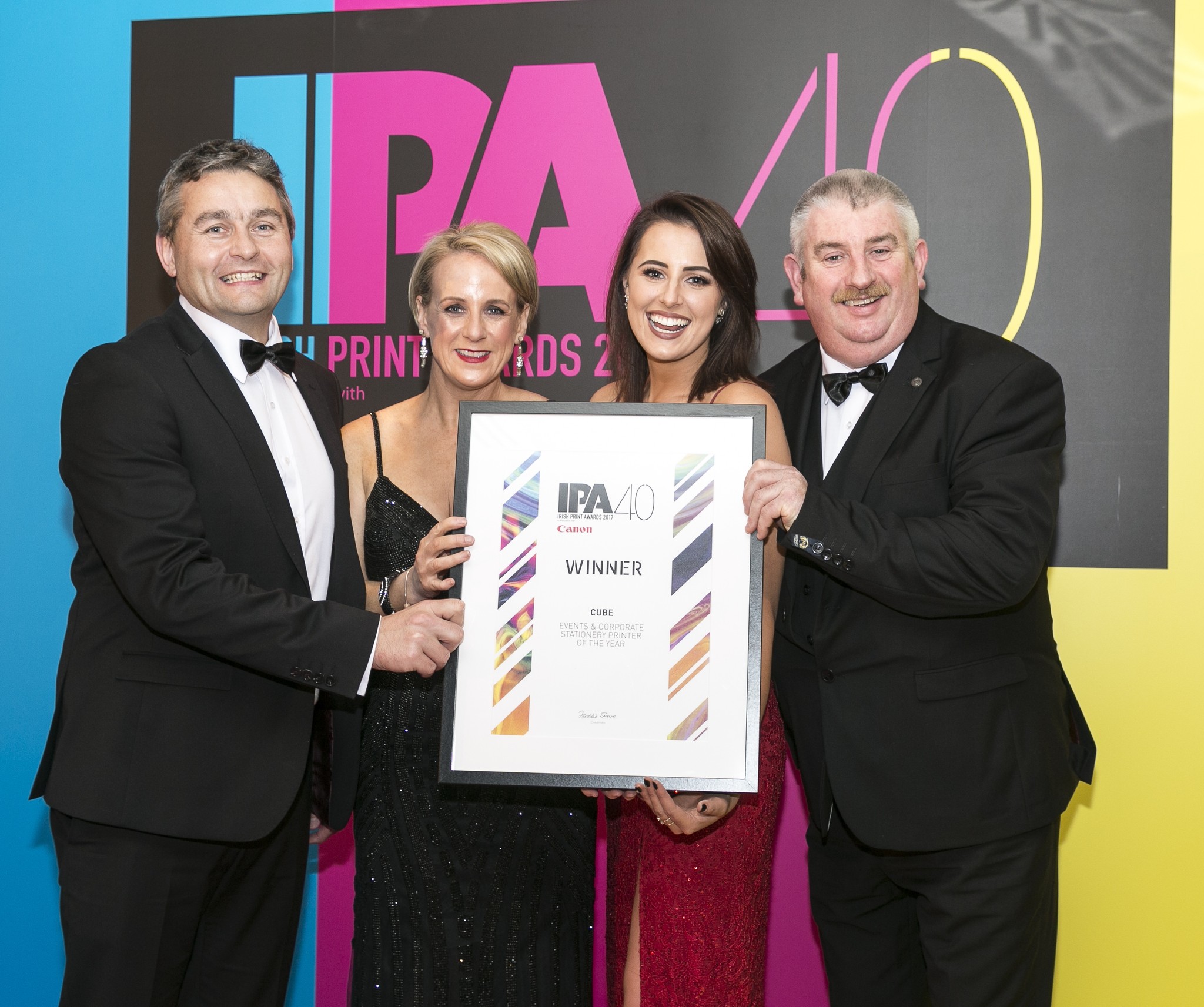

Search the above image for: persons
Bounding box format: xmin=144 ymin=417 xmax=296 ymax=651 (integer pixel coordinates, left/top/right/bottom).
xmin=26 ymin=138 xmax=467 ymax=1007
xmin=586 ymin=192 xmax=802 ymax=1007
xmin=339 ymin=219 xmax=642 ymax=1007
xmin=751 ymin=167 xmax=1096 ymax=1007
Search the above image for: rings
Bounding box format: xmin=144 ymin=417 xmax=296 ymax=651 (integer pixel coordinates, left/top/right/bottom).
xmin=656 ymin=816 xmax=670 ymax=822
xmin=660 ymin=820 xmax=674 ymax=826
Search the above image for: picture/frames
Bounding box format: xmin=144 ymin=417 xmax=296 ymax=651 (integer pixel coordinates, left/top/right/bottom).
xmin=437 ymin=401 xmax=763 ymax=797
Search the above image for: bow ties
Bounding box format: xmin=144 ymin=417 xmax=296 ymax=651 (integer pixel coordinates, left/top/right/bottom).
xmin=822 ymin=362 xmax=889 ymax=407
xmin=239 ymin=339 xmax=296 ymax=376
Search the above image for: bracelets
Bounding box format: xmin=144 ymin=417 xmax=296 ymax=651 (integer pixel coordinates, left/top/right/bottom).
xmin=404 ymin=566 xmax=414 ymax=609
xmin=378 ymin=568 xmax=406 ymax=616
